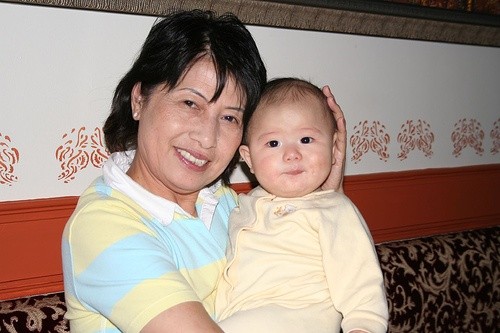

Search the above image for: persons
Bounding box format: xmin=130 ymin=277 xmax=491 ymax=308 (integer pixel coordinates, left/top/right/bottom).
xmin=215 ymin=77 xmax=388 ymax=333
xmin=61 ymin=9 xmax=346 ymax=333
